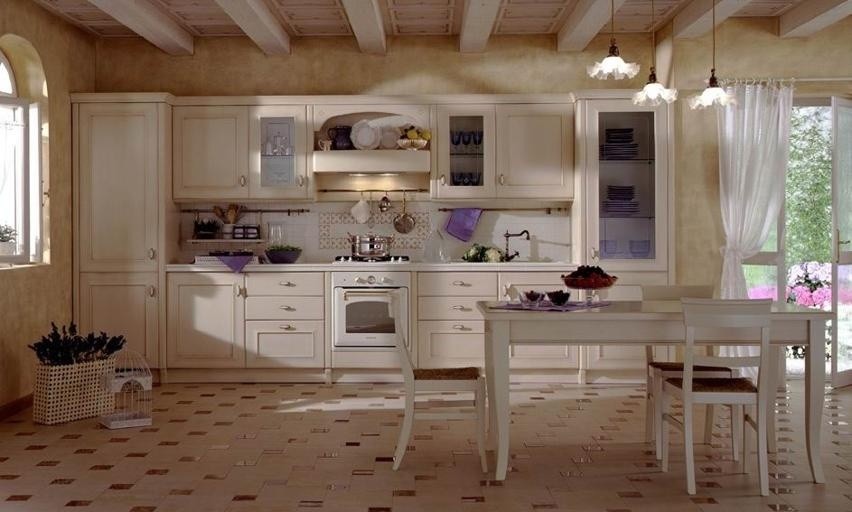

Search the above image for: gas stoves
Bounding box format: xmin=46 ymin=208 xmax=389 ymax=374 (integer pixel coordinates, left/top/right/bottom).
xmin=331 ymin=253 xmax=411 ymax=265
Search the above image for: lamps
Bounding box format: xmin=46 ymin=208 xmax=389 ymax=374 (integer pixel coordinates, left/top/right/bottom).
xmin=632 ymin=0 xmax=678 ymax=110
xmin=688 ymin=0 xmax=736 ymax=111
xmin=584 ymin=0 xmax=641 ymax=81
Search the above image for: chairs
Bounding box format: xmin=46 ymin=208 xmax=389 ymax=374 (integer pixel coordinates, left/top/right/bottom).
xmin=642 ymin=286 xmax=742 ymax=463
xmin=660 ymin=296 xmax=774 ymax=496
xmin=390 ymin=306 xmax=490 ymax=472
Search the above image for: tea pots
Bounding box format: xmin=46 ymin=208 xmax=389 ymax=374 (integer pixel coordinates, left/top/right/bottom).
xmin=319 ymin=139 xmax=332 ymax=152
xmin=327 ymin=125 xmax=354 ymax=150
xmin=261 ymin=131 xmax=294 ymax=156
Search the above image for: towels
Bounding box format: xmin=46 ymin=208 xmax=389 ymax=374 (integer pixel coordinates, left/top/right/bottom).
xmin=444 ymin=207 xmax=483 ymax=244
xmin=218 ymin=255 xmax=252 ymax=271
xmin=488 ymin=300 xmax=613 ymax=311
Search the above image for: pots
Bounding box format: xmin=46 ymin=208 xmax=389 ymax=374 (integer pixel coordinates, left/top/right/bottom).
xmin=394 ymin=194 xmax=415 ymax=234
xmin=346 ymin=230 xmax=397 ymax=258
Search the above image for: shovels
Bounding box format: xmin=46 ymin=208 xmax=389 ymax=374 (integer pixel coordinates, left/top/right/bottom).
xmin=212 ymin=204 xmax=239 ymax=224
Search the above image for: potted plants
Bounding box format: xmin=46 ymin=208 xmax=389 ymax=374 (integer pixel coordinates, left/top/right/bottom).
xmin=0 ymin=224 xmax=18 ymax=256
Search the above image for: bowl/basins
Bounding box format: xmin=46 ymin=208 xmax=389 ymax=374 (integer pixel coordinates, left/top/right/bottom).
xmin=265 ymin=248 xmax=301 ymax=264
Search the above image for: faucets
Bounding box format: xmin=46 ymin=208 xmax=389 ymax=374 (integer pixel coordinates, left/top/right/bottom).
xmin=505 ymin=230 xmax=531 ymax=241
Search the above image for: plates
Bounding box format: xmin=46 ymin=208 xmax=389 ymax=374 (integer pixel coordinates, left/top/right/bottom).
xmin=600 ymin=128 xmax=641 ymax=159
xmin=349 ymin=118 xmax=381 ymax=149
xmin=600 ymin=185 xmax=641 ymax=215
xmin=378 ymin=124 xmax=401 ymax=149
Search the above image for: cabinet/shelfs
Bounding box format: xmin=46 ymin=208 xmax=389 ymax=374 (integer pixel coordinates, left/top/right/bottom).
xmin=417 ymin=272 xmax=578 ymax=372
xmin=430 ymin=94 xmax=575 ymax=203
xmin=69 ymin=92 xmax=173 ymax=386
xmin=172 ymin=95 xmax=316 ymax=204
xmin=311 ymin=97 xmax=436 ymax=173
xmin=572 ymin=89 xmax=668 ymax=371
xmin=165 ymin=271 xmax=325 ymax=382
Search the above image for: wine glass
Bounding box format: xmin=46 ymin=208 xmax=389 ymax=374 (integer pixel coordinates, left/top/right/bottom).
xmin=472 ymin=127 xmax=483 ymax=155
xmin=450 ymin=129 xmax=463 ymax=153
xmin=461 ymin=131 xmax=474 ymax=154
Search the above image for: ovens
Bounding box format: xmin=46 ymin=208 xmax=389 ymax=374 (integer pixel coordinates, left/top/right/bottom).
xmin=331 ymin=272 xmax=412 ymax=352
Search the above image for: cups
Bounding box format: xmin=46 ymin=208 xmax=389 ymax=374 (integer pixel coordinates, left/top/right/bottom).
xmin=451 ymin=168 xmax=462 ymax=185
xmin=546 ymin=291 xmax=572 ymax=306
xmin=519 ymin=290 xmax=545 ymax=307
xmin=220 ymin=223 xmax=234 ymax=240
xmin=629 ymin=240 xmax=650 ymax=258
xmin=600 ymin=239 xmax=617 ymax=259
xmin=470 ymin=168 xmax=481 ymax=185
xmin=462 ymin=168 xmax=473 ymax=185
